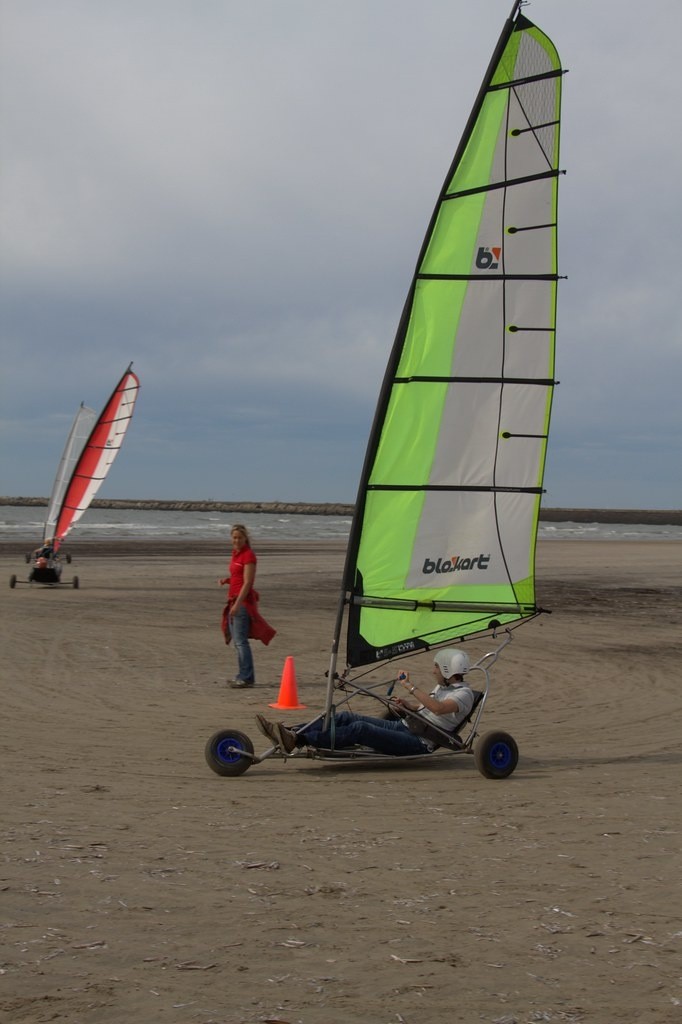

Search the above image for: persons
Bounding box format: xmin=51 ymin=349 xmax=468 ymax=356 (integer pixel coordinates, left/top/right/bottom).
xmin=34 ymin=538 xmax=60 ymax=564
xmin=216 ymin=522 xmax=272 ymax=687
xmin=255 ymin=648 xmax=475 ymax=757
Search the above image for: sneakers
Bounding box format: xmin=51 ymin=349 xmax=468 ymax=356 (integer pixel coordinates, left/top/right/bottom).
xmin=272 ymin=721 xmax=296 ymax=755
xmin=253 ymin=713 xmax=280 ymax=747
xmin=226 ymin=678 xmax=255 ymax=688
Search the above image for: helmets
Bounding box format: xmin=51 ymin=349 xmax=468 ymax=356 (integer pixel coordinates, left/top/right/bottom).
xmin=433 ymin=648 xmax=472 ymax=680
xmin=37 ymin=556 xmax=48 ymax=569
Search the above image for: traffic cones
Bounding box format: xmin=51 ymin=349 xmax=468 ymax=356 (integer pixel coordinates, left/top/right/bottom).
xmin=266 ymin=654 xmax=309 ymax=710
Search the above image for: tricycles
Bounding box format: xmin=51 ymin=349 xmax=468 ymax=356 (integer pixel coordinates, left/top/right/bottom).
xmin=199 ymin=4 xmax=568 ymax=783
xmin=9 ymin=359 xmax=142 ymax=587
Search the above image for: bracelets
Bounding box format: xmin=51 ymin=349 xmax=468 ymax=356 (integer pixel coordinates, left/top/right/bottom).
xmin=408 ymin=684 xmax=418 ymax=695
